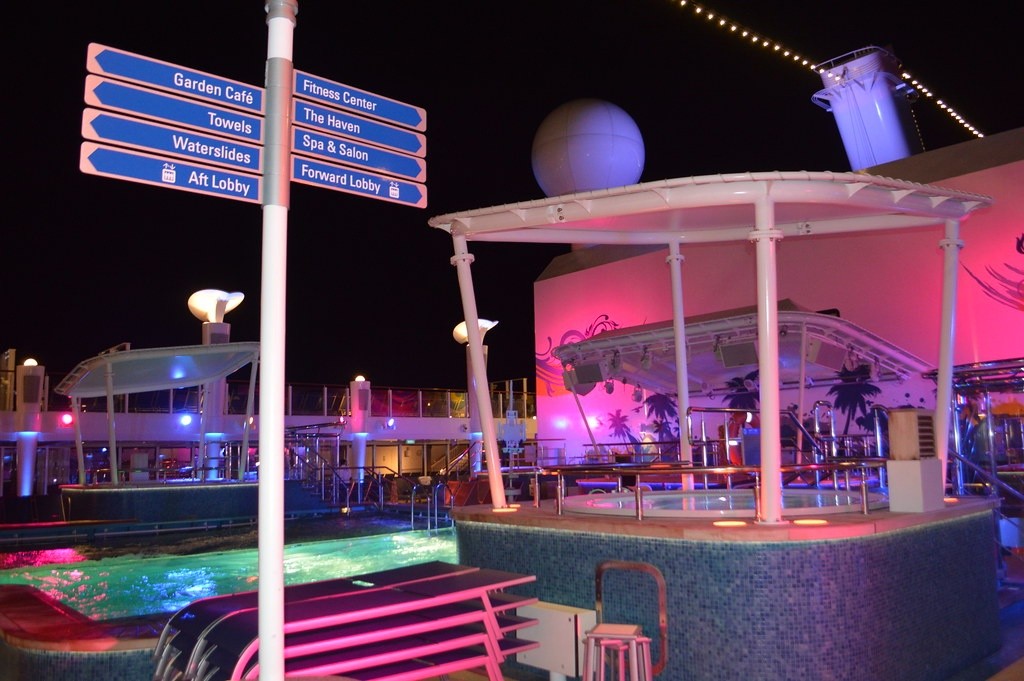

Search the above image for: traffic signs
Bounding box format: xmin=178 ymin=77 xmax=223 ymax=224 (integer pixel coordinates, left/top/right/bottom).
xmin=291 ymin=71 xmax=429 ymax=208
xmin=78 ymin=41 xmax=263 ymax=204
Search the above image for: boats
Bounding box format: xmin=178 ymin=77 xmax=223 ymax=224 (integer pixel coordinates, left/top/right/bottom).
xmin=0 ymin=286 xmax=535 ymax=547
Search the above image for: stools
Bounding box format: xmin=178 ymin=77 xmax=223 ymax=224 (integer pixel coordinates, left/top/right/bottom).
xmin=580 ymin=621 xmax=654 ymax=681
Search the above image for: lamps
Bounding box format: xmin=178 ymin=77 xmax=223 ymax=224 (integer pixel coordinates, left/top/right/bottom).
xmin=640 ymin=346 xmax=654 ymax=370
xmin=610 ymin=350 xmax=623 ymax=374
xmin=713 ymin=334 xmax=724 ymax=361
xmin=381 ymin=416 xmax=397 ymax=430
xmin=842 ymin=343 xmax=882 ymax=382
